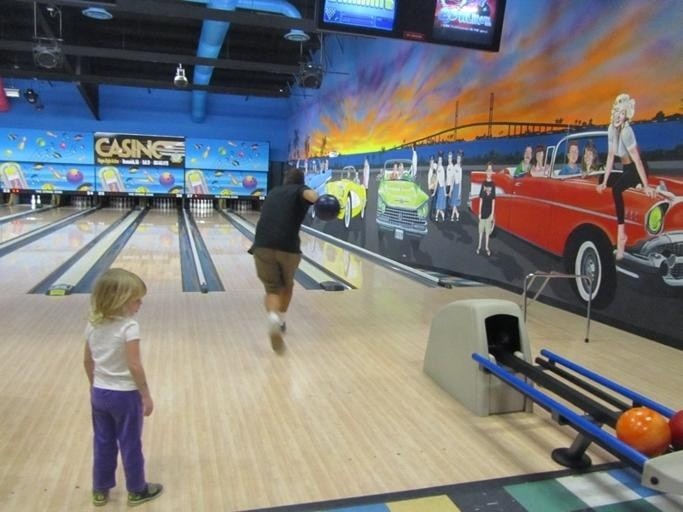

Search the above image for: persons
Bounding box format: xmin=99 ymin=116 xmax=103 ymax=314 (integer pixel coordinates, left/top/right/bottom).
xmin=528 ymin=145 xmax=552 ymax=178
xmin=80 ymin=267 xmax=166 ymax=509
xmin=250 ymin=169 xmax=339 ymax=361
xmin=513 ymin=145 xmax=532 ymax=179
xmin=304 ymin=140 xmax=464 ymax=225
xmin=558 ymin=141 xmax=583 ymax=176
xmin=580 ymin=137 xmax=602 ymax=174
xmin=474 ymin=164 xmax=496 ymax=260
xmin=595 ymin=92 xmax=659 ymax=260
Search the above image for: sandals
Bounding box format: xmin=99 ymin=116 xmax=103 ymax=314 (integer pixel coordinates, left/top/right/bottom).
xmin=475 ymin=246 xmax=481 ymax=255
xmin=484 ymin=247 xmax=491 ymax=256
xmin=430 ymin=211 xmax=460 ymax=223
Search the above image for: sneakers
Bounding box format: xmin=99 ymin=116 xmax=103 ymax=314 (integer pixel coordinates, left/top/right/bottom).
xmin=93 ymin=490 xmax=109 ymax=506
xmin=127 ymin=482 xmax=164 ymax=506
xmin=269 ymin=320 xmax=286 ymax=356
xmin=279 ymin=320 xmax=287 ymax=334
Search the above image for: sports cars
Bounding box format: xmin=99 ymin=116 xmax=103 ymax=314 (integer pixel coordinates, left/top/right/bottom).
xmin=375 ymin=157 xmax=433 ymax=249
xmin=288 ymin=153 xmax=334 ymax=221
xmin=310 ymin=164 xmax=368 ymax=229
xmin=466 ymin=123 xmax=683 ymax=309
